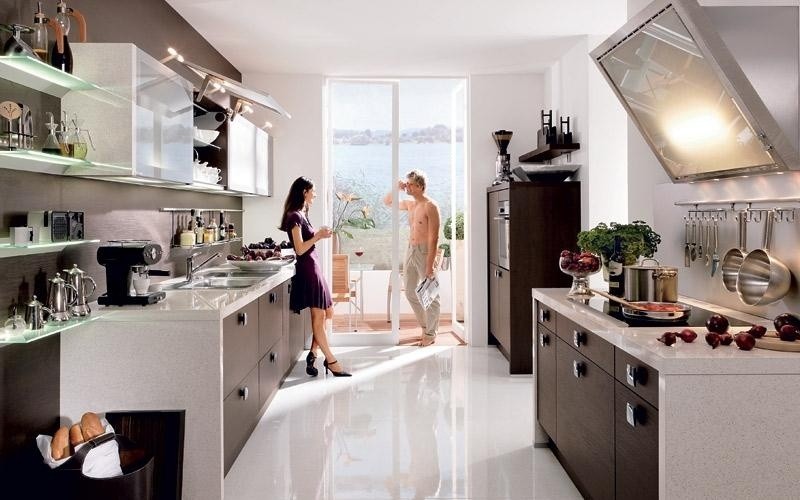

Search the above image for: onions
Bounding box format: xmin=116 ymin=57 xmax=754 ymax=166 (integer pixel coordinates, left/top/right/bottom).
xmin=227 ymin=238 xmax=294 ymax=260
xmin=560 ymin=250 xmax=599 ymax=271
xmin=658 ymin=314 xmax=800 ymax=350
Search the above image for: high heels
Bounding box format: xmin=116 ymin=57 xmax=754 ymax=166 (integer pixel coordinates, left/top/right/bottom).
xmin=323 ymin=359 xmax=353 ymax=378
xmin=306 ymin=351 xmax=319 ymax=379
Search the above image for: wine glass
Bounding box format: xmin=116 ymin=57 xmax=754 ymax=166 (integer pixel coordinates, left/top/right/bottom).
xmin=352 ymin=246 xmax=366 ymax=266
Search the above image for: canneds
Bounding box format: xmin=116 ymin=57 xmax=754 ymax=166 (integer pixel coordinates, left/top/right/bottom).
xmin=180 ymin=230 xmax=196 ymax=248
xmin=204 ymin=229 xmax=214 ymax=243
xmin=196 ymin=227 xmax=203 ymax=244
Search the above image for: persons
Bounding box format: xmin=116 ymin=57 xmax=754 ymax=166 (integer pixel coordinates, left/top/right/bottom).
xmin=278 ymin=175 xmax=353 ymax=377
xmin=385 ymin=171 xmax=442 ymax=346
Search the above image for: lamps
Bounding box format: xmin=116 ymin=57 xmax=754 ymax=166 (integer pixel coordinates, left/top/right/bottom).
xmin=158 ymin=45 xmax=274 ymax=132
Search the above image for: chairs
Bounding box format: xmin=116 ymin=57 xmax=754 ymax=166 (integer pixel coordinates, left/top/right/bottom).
xmin=332 ymin=254 xmax=358 ymax=333
xmin=387 ymin=248 xmax=445 ymax=331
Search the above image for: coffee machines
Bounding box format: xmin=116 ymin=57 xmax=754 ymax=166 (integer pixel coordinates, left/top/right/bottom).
xmin=93 ymin=244 xmax=167 ymax=307
xmin=490 ymin=129 xmax=513 ymax=185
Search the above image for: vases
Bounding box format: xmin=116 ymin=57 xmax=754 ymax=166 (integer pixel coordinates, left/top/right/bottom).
xmin=333 ymin=233 xmax=340 ymax=254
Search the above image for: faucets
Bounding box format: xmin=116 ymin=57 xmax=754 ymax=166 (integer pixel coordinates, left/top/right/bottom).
xmin=186 ymin=251 xmax=223 ymax=282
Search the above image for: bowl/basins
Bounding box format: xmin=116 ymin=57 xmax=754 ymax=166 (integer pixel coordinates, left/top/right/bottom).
xmin=194 ymin=127 xmax=222 ymax=145
xmin=225 ymin=258 xmax=294 ymax=271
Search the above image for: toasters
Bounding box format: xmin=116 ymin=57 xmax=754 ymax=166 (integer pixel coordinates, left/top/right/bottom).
xmin=173 ymin=209 xmax=236 ymax=247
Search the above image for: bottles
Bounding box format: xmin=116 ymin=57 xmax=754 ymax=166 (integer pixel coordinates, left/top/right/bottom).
xmin=4 ymin=316 xmax=26 ymax=337
xmin=41 ymin=106 xmax=96 ymax=160
xmin=30 ymin=0 xmax=88 ymax=76
xmin=607 ymin=234 xmax=626 ymax=306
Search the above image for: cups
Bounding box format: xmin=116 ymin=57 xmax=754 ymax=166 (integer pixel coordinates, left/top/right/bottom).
xmin=190 ymin=160 xmax=224 ymax=185
xmin=133 ymin=279 xmax=150 ymax=295
xmin=149 ymin=284 xmax=162 ymax=292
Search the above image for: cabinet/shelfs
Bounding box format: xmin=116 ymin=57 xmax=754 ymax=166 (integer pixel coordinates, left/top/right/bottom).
xmin=614 ymin=345 xmax=799 ymax=499
xmin=286 ymin=274 xmax=314 ymax=373
xmin=260 ymin=285 xmax=287 ymax=409
xmin=58 ymin=299 xmax=259 ymax=480
xmin=179 ymin=56 xmax=291 ymax=193
xmin=486 ymin=180 xmax=582 ymax=374
xmin=536 ymin=298 xmax=556 ymax=447
xmin=60 ymin=41 xmax=191 ymax=188
xmin=227 ymin=109 xmax=274 ymax=198
xmin=0 ymin=239 xmax=98 ymax=348
xmin=0 ymin=56 xmax=98 ymax=174
xmin=556 ymin=316 xmax=614 ymax=499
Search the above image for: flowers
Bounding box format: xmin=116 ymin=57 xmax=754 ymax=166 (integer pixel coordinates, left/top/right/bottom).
xmin=334 ymin=192 xmax=375 ymax=240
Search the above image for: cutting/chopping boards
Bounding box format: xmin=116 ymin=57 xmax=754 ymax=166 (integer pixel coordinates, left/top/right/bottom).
xmin=746 ymin=331 xmax=800 ymax=352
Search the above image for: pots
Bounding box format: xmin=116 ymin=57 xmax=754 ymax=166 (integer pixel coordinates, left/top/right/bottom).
xmin=620 ymin=258 xmax=679 ymax=303
xmin=620 ymin=301 xmax=691 ymax=320
xmin=721 ymin=209 xmax=792 ymax=306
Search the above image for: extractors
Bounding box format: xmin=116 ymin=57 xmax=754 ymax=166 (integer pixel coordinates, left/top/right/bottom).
xmin=578 ymin=1 xmax=800 ymax=188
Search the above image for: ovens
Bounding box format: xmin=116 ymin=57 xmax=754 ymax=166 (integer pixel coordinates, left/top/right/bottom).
xmin=494 ymin=200 xmax=510 ymax=270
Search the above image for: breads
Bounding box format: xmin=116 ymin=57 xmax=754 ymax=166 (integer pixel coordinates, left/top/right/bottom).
xmin=69 ymin=424 xmax=84 ymax=456
xmin=81 ymin=412 xmax=106 ymax=443
xmin=52 ymin=426 xmax=70 ymax=460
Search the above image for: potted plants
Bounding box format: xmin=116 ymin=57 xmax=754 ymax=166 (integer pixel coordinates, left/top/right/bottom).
xmin=577 ymin=219 xmax=661 ymax=283
xmin=439 ymin=244 xmax=450 ymax=271
xmin=444 ymin=212 xmax=466 ymax=259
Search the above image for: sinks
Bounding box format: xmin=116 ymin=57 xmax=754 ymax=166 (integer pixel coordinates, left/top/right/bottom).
xmin=198 ymin=268 xmax=279 ymax=279
xmin=165 ymin=278 xmax=271 ymax=291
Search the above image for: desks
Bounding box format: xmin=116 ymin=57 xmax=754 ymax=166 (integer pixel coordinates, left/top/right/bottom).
xmin=0 ymin=433 xmax=160 ymax=500
xmin=348 ymin=263 xmax=375 ymax=332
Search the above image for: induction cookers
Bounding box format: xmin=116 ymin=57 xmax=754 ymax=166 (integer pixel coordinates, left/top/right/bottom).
xmin=570 ymin=297 xmax=753 ymax=328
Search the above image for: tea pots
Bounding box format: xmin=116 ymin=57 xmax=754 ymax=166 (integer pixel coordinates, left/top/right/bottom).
xmin=23 ymin=294 xmax=51 ymax=330
xmin=61 ymin=262 xmax=97 ymax=316
xmin=45 ymin=273 xmax=78 ymax=322
